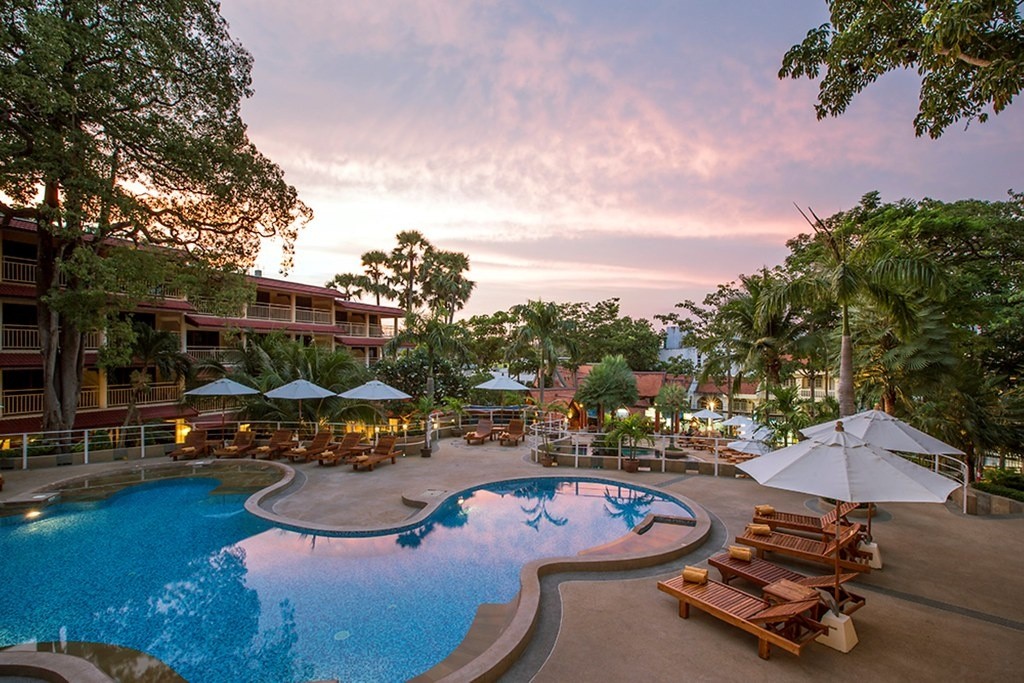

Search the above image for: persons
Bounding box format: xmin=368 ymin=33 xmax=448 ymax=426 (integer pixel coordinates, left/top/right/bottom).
xmin=681 ymin=415 xmax=705 ymax=443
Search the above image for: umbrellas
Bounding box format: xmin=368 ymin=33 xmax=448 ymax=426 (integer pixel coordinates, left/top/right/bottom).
xmin=734 ymin=422 xmax=962 ymax=617
xmin=475 ymin=375 xmax=530 ymax=427
xmin=691 ymin=408 xmax=723 ymax=431
xmin=263 ymin=375 xmax=337 ymax=441
xmin=337 ymin=377 xmax=413 ymax=449
xmin=183 ymin=374 xmax=260 ymax=442
xmin=722 ymin=415 xmax=775 ymax=455
xmin=798 ymin=406 xmax=967 ymax=543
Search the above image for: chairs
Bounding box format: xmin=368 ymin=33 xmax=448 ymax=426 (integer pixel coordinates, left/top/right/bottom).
xmin=343 ymin=435 xmax=403 ymax=472
xmin=247 ymin=429 xmax=295 ymax=461
xmin=677 ymin=438 xmax=759 ymax=464
xmin=463 ymin=420 xmax=494 ymax=444
xmin=169 ymin=430 xmax=211 ymax=462
xmin=283 ymin=431 xmax=339 ymax=463
xmin=214 ymin=430 xmax=258 ymax=459
xmin=657 ymin=502 xmax=872 ymax=660
xmin=499 ymin=419 xmax=527 ymax=446
xmin=311 ymin=432 xmax=365 ymax=467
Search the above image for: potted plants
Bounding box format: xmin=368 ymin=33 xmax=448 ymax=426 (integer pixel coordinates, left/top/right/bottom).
xmin=520 ymin=395 xmax=571 ymax=467
xmin=440 ymin=396 xmax=472 ymax=437
xmin=600 ymin=410 xmax=656 ymax=473
xmin=405 ymin=394 xmax=442 ymax=458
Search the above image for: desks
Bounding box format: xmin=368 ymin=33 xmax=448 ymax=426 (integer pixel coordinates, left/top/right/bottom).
xmin=278 ymin=440 xmax=301 ymax=457
xmin=204 ymin=440 xmax=225 ymax=458
xmin=346 ymin=446 xmax=374 ymax=458
xmin=492 ymin=427 xmax=506 ymax=441
